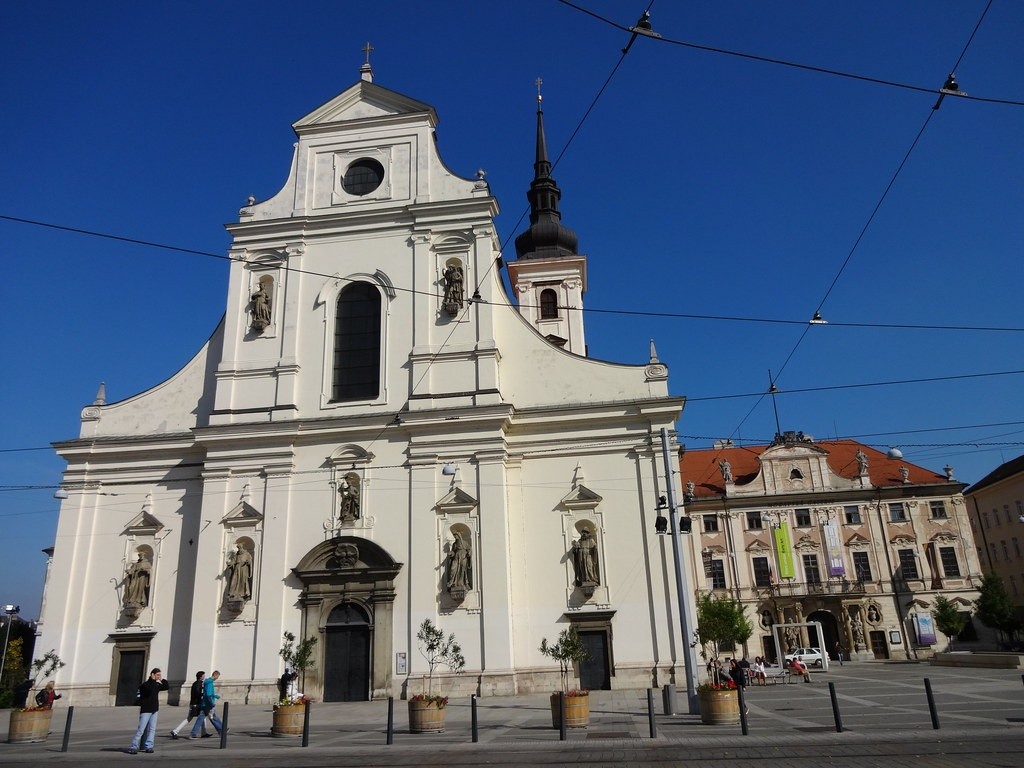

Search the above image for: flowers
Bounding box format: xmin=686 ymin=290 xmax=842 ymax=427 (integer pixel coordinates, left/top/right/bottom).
xmin=277 ymin=696 xmax=316 ymax=706
xmin=564 ymin=688 xmax=590 ymax=698
xmin=17 ymin=705 xmax=50 ymax=713
xmin=696 ymin=679 xmax=739 ymax=694
xmin=409 ymin=694 xmax=449 ymax=709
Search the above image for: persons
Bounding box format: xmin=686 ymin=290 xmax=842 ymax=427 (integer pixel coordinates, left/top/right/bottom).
xmin=753 ymin=656 xmax=771 ymax=685
xmin=856 ymin=448 xmax=869 ymax=473
xmin=226 ymin=543 xmax=253 ymax=598
xmin=738 ymin=656 xmax=754 ymax=683
xmin=170 ymin=669 xmax=230 ymax=739
xmin=786 ymin=617 xmax=800 ymax=651
xmin=35 ymin=680 xmax=62 ymax=734
xmin=446 ymin=532 xmax=472 ymax=586
xmin=279 ymin=668 xmax=298 ymax=701
xmin=125 ymin=553 xmax=152 ymax=605
xmin=252 ymin=282 xmax=271 ymax=322
xmin=729 ymin=659 xmax=749 ymax=716
xmin=340 ymin=480 xmax=359 ymax=518
xmin=851 ymin=613 xmax=865 ymax=645
xmin=834 ymin=641 xmax=846 ymax=667
xmin=572 ymin=526 xmax=599 ymax=582
xmin=713 ymin=660 xmax=731 ymax=685
xmin=442 ymin=264 xmax=464 ymax=303
xmin=791 ymin=656 xmax=812 ymax=682
xmin=719 ymin=458 xmax=733 ymax=482
xmin=130 ymin=667 xmax=169 ymax=753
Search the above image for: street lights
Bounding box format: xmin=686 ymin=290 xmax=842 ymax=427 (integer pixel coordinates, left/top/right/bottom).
xmin=0 ymin=604 xmax=20 ymax=684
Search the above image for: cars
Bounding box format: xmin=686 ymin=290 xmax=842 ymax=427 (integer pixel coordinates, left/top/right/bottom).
xmin=774 ymin=647 xmax=831 ymax=666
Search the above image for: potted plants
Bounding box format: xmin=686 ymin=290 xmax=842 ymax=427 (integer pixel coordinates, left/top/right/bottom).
xmin=6 ymin=648 xmax=67 ymax=749
xmin=261 ymin=629 xmax=321 ymax=739
xmin=536 ymin=621 xmax=596 ymax=730
xmin=689 ymin=586 xmax=756 ymax=730
xmin=406 ymin=616 xmax=467 ymax=736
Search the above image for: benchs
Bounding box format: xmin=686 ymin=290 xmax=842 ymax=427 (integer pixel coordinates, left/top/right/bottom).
xmin=740 ymin=667 xmax=753 ymax=688
xmin=787 ymin=666 xmax=807 ymax=684
xmin=755 ymin=668 xmax=787 ymax=685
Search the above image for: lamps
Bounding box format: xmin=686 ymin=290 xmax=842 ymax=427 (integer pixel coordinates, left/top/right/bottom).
xmin=655 ymin=495 xmax=668 ymax=508
xmin=652 ymin=516 xmax=671 ymax=536
xmin=678 ymin=516 xmax=694 ymax=533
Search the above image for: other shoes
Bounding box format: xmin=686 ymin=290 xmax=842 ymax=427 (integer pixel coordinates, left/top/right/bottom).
xmin=218 ymin=728 xmax=231 ymax=735
xmin=138 ymin=746 xmax=155 ymax=753
xmin=201 ymin=733 xmax=213 ymax=738
xmin=170 ymin=730 xmax=179 ymax=739
xmin=189 ymin=736 xmax=199 ymax=739
xmin=126 ymin=748 xmax=138 ymax=754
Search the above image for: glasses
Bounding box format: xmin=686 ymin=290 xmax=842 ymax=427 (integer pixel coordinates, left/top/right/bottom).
xmin=156 ymin=673 xmax=161 ymax=676
